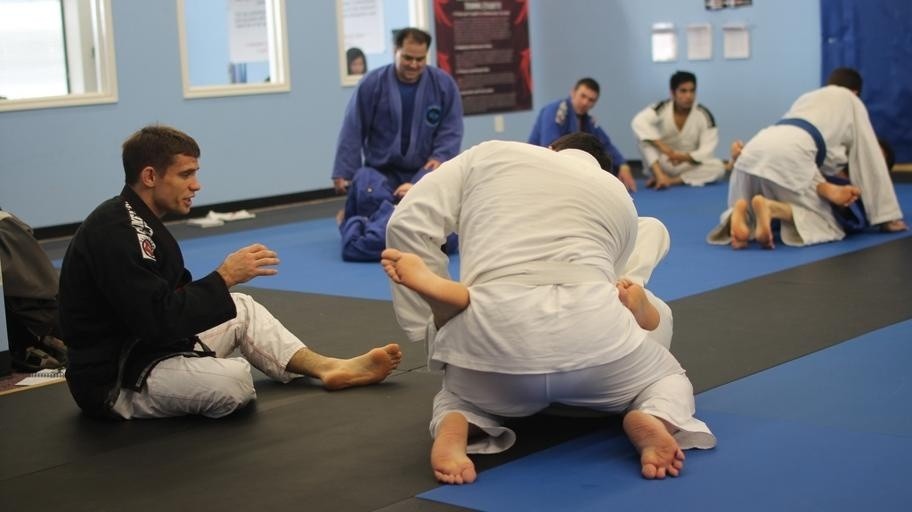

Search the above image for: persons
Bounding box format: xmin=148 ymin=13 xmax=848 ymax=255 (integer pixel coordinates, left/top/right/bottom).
xmin=59 ymin=127 xmax=403 ymax=420
xmin=703 ymin=67 xmax=909 ymax=251
xmin=629 ymin=71 xmax=726 ymax=190
xmin=527 ymin=77 xmax=637 ymax=193
xmin=332 ymin=27 xmax=464 ymax=193
xmin=386 ymin=131 xmax=718 ymax=487
xmin=1 ymin=206 xmax=69 ymax=368
xmin=376 ymin=216 xmax=681 ymax=352
xmin=347 ymin=48 xmax=367 ymax=76
xmin=336 ymin=166 xmax=461 ymax=261
xmin=732 ymin=135 xmax=896 ymax=235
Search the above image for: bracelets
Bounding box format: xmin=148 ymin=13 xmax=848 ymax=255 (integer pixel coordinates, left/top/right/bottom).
xmin=619 ymin=164 xmax=631 ymax=170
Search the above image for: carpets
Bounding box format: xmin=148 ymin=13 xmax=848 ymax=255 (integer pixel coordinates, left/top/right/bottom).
xmin=50 ymin=159 xmax=910 ymax=310
xmin=417 ymin=316 xmax=912 ymax=510
